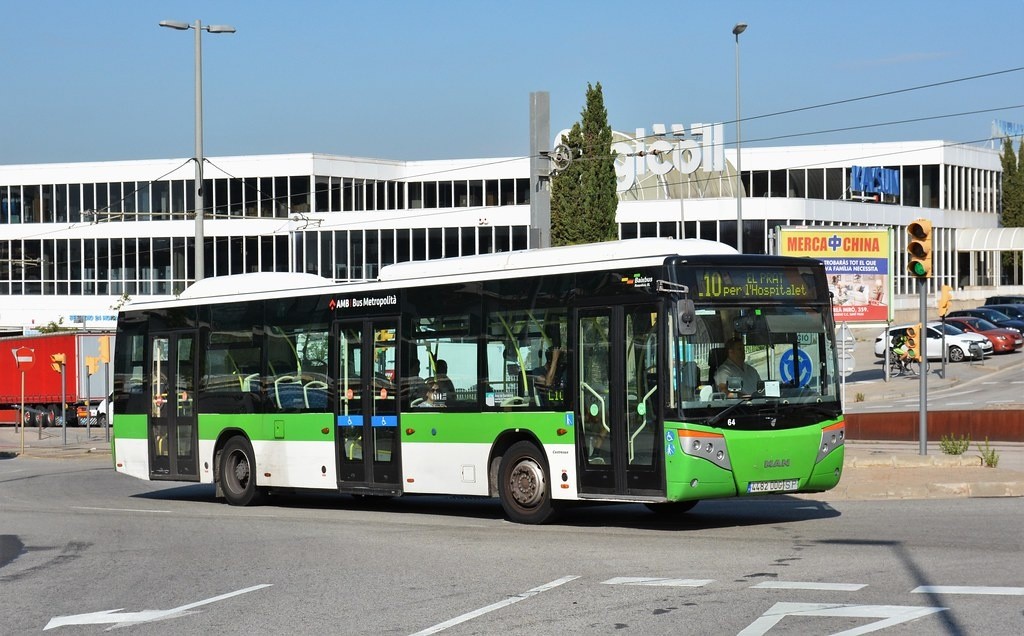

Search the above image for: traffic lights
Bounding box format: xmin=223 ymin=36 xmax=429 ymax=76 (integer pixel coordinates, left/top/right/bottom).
xmin=51 ymin=363 xmax=62 ymax=373
xmin=906 ymin=218 xmax=932 ymax=278
xmin=906 ymin=325 xmax=920 ymax=359
xmin=939 ymin=286 xmax=952 ymax=317
xmin=86 ymin=357 xmax=99 ymax=375
xmin=374 ymin=329 xmax=394 ymax=352
xmin=98 ymin=336 xmax=110 ymax=363
xmin=50 ymin=353 xmax=66 ymax=365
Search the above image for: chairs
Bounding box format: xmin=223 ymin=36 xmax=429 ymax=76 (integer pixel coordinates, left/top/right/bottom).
xmin=233 ymin=368 xmax=436 ymax=412
xmin=125 ymin=371 xmax=191 ymax=393
xmin=502 ymin=395 xmax=530 ymax=408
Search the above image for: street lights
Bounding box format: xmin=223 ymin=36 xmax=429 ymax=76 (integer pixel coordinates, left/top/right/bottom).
xmin=733 ymin=23 xmax=747 ymax=253
xmin=159 ymin=19 xmax=236 ymax=282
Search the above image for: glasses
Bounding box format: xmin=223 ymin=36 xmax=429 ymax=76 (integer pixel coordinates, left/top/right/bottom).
xmin=429 ymin=388 xmax=440 ymax=393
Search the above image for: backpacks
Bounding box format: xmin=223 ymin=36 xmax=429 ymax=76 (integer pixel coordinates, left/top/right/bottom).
xmin=891 ymin=335 xmax=903 ymax=345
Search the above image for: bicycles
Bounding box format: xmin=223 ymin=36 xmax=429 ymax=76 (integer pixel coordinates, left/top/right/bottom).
xmin=882 ymin=351 xmax=930 ymax=377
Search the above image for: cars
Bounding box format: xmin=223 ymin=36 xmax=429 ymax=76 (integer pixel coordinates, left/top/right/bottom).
xmin=946 ymin=309 xmax=1024 ymax=338
xmin=875 ymin=321 xmax=994 ymax=362
xmin=96 ymin=383 xmax=193 ymax=427
xmin=984 ymin=295 xmax=1024 ymax=306
xmin=933 ymin=317 xmax=1024 ymax=353
xmin=977 ymin=304 xmax=1024 ymax=321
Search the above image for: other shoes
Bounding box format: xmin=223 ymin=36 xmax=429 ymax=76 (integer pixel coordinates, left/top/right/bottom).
xmin=903 ymin=366 xmax=910 ymax=371
xmin=894 ymin=360 xmax=902 ymax=366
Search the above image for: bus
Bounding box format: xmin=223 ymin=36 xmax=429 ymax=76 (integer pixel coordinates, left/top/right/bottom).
xmin=110 ymin=237 xmax=846 ymax=524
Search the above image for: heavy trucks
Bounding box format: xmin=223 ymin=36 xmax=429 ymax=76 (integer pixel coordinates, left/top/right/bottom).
xmin=0 ymin=330 xmax=136 ymax=427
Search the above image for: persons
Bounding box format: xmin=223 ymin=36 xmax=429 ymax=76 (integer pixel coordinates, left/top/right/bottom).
xmin=893 ymin=328 xmax=916 ymax=371
xmin=429 ymin=360 xmax=456 ymax=400
xmin=532 ymin=346 xmax=568 ymax=407
xmin=713 ymin=337 xmax=763 ymax=398
xmin=418 ymin=382 xmax=447 ymax=407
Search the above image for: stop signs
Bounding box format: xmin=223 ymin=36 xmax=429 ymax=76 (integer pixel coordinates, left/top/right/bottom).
xmin=15 ymin=347 xmax=35 ymax=371
